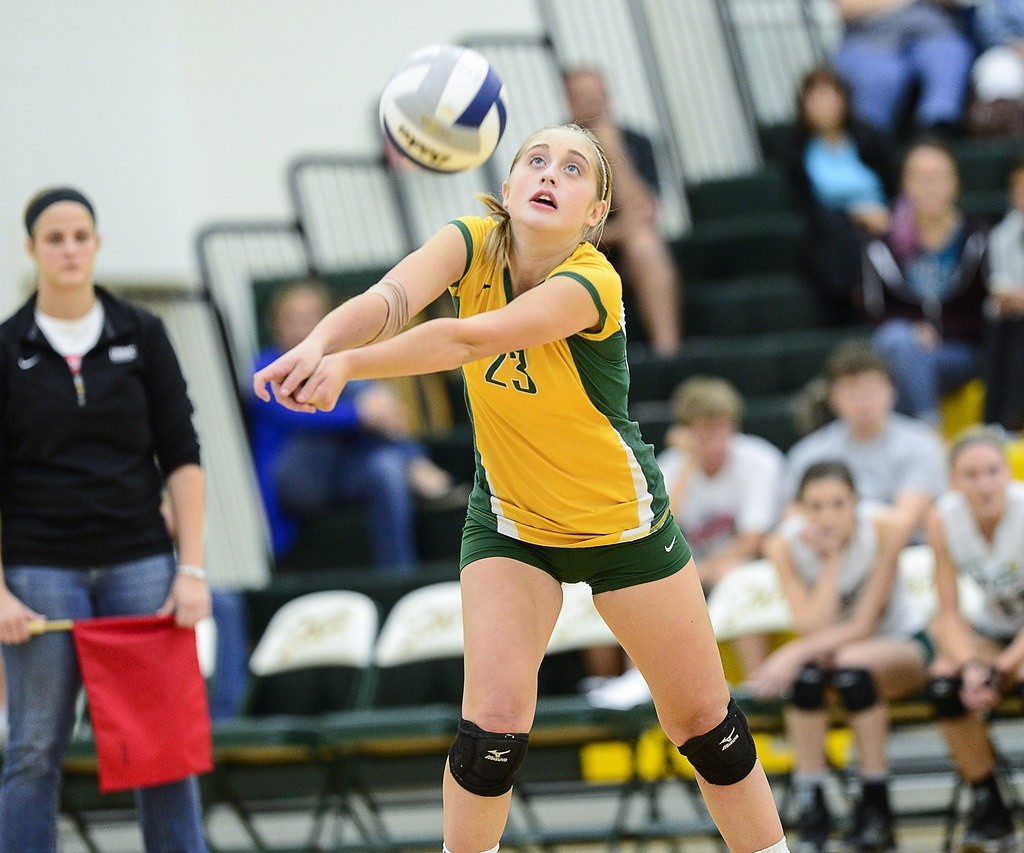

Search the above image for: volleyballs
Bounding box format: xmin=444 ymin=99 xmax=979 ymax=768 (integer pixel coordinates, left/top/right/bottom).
xmin=379 ymin=43 xmax=510 ymax=176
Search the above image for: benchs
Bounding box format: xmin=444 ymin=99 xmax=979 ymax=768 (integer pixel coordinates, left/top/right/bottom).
xmin=239 ymin=120 xmax=1024 ymax=713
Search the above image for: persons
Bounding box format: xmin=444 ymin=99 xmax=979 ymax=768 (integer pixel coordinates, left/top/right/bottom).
xmin=923 ymin=434 xmax=1024 ymax=853
xmin=750 ymin=459 xmax=936 ymax=853
xmin=252 ymin=120 xmax=789 ymax=853
xmin=776 ymin=345 xmax=945 ymax=552
xmin=785 ymin=0 xmax=1024 ymax=431
xmin=250 ymin=280 xmax=471 ymax=580
xmin=0 ymin=186 xmax=213 ymax=853
xmin=567 ymin=64 xmax=684 ymax=362
xmin=653 ymin=375 xmax=786 ymax=673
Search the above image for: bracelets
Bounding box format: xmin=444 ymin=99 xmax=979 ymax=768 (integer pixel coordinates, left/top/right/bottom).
xmin=176 ymin=562 xmax=205 ymax=581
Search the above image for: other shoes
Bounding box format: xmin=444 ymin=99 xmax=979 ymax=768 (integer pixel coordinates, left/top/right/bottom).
xmin=842 ymin=800 xmax=898 ymax=853
xmin=963 ymin=777 xmax=1018 ymax=853
xmin=795 ymin=785 xmax=831 ymax=853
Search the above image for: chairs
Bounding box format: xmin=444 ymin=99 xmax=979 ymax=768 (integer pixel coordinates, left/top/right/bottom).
xmin=59 ymin=546 xmax=1024 ymax=853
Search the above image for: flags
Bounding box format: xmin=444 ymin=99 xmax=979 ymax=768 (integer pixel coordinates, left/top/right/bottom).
xmin=72 ymin=612 xmax=212 ymax=791
